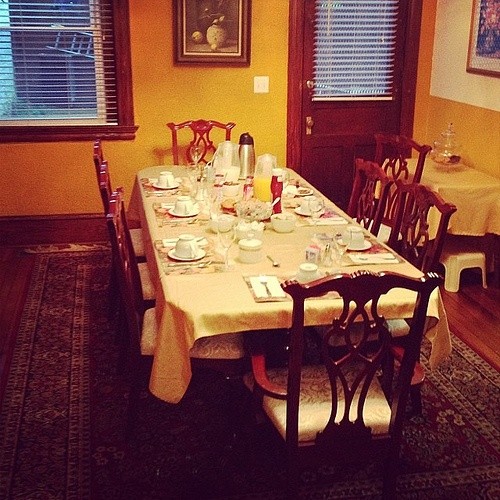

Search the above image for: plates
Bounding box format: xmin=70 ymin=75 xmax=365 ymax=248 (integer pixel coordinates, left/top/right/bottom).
xmin=152 ymin=182 xmax=180 ymax=190
xmin=169 ymin=208 xmax=200 ymax=217
xmin=288 ymin=185 xmax=313 ymax=195
xmin=168 ymin=249 xmax=205 ymax=261
xmin=294 ymin=207 xmax=327 ymax=217
xmin=339 ymin=238 xmax=372 ymax=251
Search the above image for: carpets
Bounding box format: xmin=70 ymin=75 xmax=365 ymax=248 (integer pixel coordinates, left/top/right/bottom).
xmin=0 ymin=254 xmax=500 ymax=500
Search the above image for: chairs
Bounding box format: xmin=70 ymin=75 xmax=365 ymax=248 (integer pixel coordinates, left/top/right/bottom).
xmin=93 ymin=122 xmax=457 ymax=500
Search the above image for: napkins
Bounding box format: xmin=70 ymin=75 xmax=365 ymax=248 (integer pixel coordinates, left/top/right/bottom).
xmin=251 ymin=276 xmax=286 ymax=298
xmin=349 ymin=254 xmax=399 ymax=264
xmin=161 ymin=202 xmax=199 ymax=208
xmin=311 ymin=218 xmax=348 ymax=225
xmin=163 ymin=237 xmax=210 ymax=246
xmin=149 ymin=177 xmax=183 ymax=183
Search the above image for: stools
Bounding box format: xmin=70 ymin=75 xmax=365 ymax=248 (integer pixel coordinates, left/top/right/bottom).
xmin=437 ymin=242 xmax=487 ymax=292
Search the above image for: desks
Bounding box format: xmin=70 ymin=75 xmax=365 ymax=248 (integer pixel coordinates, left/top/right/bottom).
xmin=377 ymin=157 xmax=500 ymax=237
xmin=138 ymin=168 xmax=453 ymax=403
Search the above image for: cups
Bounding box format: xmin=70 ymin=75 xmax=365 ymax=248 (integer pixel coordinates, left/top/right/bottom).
xmin=342 ymin=226 xmax=365 ymax=248
xmin=157 ymin=171 xmax=174 ymax=187
xmin=174 ymin=196 xmax=194 ymax=215
xmin=300 ymin=195 xmax=324 ymax=213
xmin=175 ymin=236 xmax=199 ymax=259
xmin=283 ymin=171 xmax=290 ymax=189
xmin=297 ymin=263 xmax=319 ymax=285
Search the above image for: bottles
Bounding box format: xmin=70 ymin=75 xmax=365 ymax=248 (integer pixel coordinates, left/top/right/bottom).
xmin=215 ymin=143 xmax=283 ymax=214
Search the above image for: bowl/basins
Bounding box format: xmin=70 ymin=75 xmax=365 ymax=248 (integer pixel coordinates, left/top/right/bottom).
xmin=210 ymin=199 xmax=296 ymax=250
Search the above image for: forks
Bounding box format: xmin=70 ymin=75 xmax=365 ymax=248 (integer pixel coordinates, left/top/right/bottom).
xmin=259 ymin=273 xmax=272 ymax=298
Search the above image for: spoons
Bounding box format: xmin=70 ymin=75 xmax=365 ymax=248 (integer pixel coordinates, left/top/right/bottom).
xmin=166 ymin=260 xmax=212 ymax=271
xmin=266 ymin=256 xmax=281 ymax=267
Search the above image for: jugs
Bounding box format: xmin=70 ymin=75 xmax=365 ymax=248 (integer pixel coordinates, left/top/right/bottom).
xmin=239 ymin=132 xmax=255 ymax=178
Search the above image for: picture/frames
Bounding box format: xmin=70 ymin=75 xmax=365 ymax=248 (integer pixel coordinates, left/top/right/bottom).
xmin=464 ymin=0 xmax=500 ymax=79
xmin=173 ymin=0 xmax=251 ymax=66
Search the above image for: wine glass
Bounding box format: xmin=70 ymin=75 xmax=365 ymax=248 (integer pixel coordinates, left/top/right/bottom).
xmin=309 ymin=200 xmax=324 ymax=245
xmin=190 ymin=145 xmax=204 ymax=164
xmin=319 ymin=233 xmax=350 ymax=278
xmin=187 ymin=162 xmax=238 ymax=273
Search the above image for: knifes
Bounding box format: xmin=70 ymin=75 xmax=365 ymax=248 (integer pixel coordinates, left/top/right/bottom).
xmin=167 ymin=260 xmax=224 ymax=267
